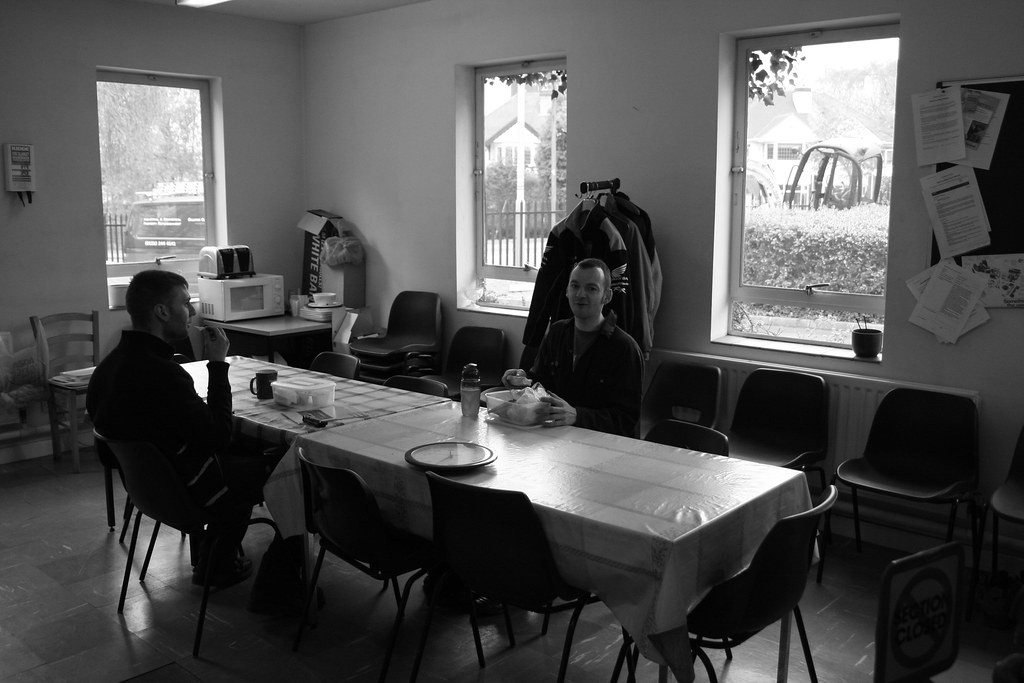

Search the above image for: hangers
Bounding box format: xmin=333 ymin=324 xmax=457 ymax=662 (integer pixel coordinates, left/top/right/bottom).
xmin=574 ymin=180 xmax=619 ymax=214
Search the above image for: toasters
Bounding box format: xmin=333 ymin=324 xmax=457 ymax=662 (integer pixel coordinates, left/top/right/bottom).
xmin=198 ymin=244 xmax=257 ymax=280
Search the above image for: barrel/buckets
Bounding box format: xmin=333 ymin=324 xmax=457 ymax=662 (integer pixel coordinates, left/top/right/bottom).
xmin=108 ymin=284 xmax=130 ymax=306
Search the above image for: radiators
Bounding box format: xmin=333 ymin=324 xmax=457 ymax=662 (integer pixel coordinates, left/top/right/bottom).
xmin=646 ymin=342 xmax=984 ymax=522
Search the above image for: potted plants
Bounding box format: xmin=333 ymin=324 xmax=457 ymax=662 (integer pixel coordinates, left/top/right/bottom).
xmin=852 ymin=329 xmax=882 ymax=357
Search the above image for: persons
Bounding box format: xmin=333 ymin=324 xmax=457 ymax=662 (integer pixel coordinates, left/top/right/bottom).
xmin=84 ymin=270 xmax=290 ymax=590
xmin=421 ymin=253 xmax=644 ymax=617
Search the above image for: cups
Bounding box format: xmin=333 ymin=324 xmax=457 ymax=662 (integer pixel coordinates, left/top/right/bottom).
xmin=250 ymin=369 xmax=278 ymax=399
xmin=288 ymin=288 xmax=303 ymax=296
xmin=289 ymin=298 xmax=309 ymax=316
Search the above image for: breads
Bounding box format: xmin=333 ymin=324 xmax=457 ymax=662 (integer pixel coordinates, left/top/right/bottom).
xmin=507 ymin=375 xmax=533 ymax=386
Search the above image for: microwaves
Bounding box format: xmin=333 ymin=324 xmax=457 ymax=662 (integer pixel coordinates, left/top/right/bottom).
xmin=199 ymin=273 xmax=285 ymax=321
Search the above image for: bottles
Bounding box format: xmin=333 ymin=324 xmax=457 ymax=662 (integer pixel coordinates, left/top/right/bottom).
xmin=458 ymin=362 xmax=481 ymax=417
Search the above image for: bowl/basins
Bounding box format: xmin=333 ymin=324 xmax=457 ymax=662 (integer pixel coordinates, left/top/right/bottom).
xmin=486 ymin=389 xmax=553 ymax=425
xmin=313 ymin=293 xmax=337 ymax=306
xmin=272 ymin=377 xmax=336 ymax=410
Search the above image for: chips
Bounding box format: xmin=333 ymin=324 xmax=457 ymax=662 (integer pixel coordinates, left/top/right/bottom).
xmin=193 ymin=326 xmax=205 ymax=332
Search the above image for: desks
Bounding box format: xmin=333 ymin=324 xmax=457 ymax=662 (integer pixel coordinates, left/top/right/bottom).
xmin=262 ymin=401 xmax=820 ymax=683
xmin=59 ymin=354 xmax=450 ymax=598
xmin=202 ymin=316 xmax=333 ymax=365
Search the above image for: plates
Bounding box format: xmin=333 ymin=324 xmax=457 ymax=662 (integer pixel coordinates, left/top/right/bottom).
xmin=299 ymin=302 xmax=354 ymax=322
xmin=404 ymin=441 xmax=499 ymax=469
xmin=476 ymin=301 xmax=529 ymax=309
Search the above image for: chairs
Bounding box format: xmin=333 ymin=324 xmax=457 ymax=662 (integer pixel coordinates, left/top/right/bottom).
xmin=722 ymin=368 xmax=830 ymax=582
xmin=411 ymin=471 xmax=637 ymax=683
xmin=818 ymin=387 xmax=1023 ymax=682
xmin=92 ymin=424 xmax=299 ymax=657
xmin=302 ymin=291 xmax=508 ymax=404
xmin=606 ymin=485 xmax=839 ymax=682
xmin=638 ymin=364 xmax=722 ymax=443
xmin=30 ymin=310 xmax=102 ymax=472
xmin=499 ymin=427 xmax=733 ymax=683
xmin=286 ymin=446 xmax=517 ymax=683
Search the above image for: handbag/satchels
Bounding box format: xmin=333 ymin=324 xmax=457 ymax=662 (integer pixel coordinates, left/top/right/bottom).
xmin=250 ymin=535 xmax=325 ymax=617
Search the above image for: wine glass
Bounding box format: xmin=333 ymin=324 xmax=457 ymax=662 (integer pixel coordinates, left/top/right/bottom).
xmin=464 ymin=287 xmax=483 ymax=308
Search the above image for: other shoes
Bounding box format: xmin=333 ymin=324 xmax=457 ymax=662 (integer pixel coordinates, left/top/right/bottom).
xmin=473 ymin=595 xmax=504 ymax=615
xmin=192 ymin=558 xmax=252 ymax=587
